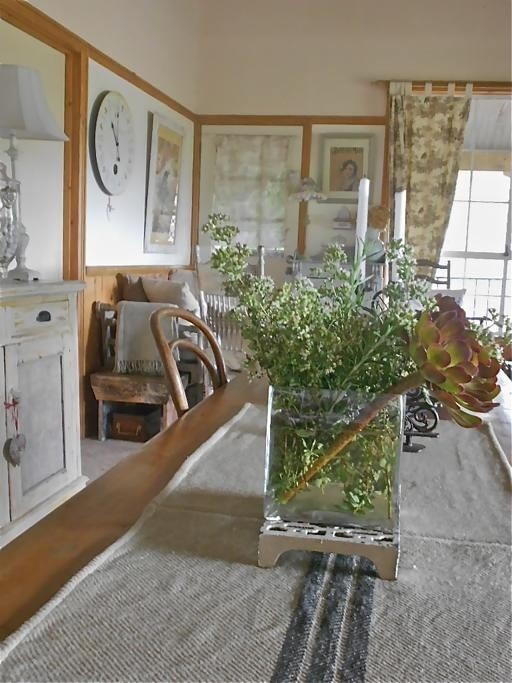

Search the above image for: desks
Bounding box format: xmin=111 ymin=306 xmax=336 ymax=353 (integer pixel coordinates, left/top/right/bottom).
xmin=1 ymin=354 xmax=512 ymax=681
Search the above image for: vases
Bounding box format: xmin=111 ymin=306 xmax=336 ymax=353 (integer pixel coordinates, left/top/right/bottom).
xmin=260 ymin=382 xmax=407 ymax=530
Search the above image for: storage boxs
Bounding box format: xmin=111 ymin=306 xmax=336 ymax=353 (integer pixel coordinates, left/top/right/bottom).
xmin=108 ymin=407 xmax=163 ymax=441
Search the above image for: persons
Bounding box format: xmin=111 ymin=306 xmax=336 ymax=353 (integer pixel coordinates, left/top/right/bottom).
xmin=338 ymin=160 xmax=360 ymax=192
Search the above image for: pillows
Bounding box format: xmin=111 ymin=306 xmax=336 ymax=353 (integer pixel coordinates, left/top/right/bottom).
xmin=120 ymin=263 xmax=203 ymax=312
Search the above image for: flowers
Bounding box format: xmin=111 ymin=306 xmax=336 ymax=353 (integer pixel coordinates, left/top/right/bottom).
xmin=193 ymin=209 xmax=511 ymax=531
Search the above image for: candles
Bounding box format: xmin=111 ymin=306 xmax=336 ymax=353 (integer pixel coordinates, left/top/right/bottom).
xmin=351 ymin=172 xmax=372 ymax=288
xmin=391 ymin=188 xmax=410 ymax=287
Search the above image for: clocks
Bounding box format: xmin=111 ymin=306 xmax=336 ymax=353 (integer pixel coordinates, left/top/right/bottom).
xmin=91 ymin=89 xmax=137 ymax=198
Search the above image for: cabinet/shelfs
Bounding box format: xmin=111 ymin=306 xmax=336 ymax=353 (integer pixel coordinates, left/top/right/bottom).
xmin=0 ymin=278 xmax=91 ymax=555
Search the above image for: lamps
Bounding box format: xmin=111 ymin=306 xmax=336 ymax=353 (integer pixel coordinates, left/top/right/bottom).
xmin=1 ymin=62 xmax=72 ymax=282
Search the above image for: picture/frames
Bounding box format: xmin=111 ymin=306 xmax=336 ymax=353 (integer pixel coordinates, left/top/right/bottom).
xmin=318 ymin=132 xmax=377 ymax=203
xmin=144 ymin=112 xmax=187 ymax=255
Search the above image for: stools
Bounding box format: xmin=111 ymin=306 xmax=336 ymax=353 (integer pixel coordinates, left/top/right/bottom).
xmin=88 ymin=371 xmax=189 ymax=445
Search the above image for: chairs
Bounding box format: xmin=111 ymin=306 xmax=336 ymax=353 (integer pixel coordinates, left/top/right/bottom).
xmin=148 ymin=305 xmax=227 ymax=418
xmin=190 ymin=243 xmax=265 ymax=398
xmin=398 ymin=253 xmax=451 ymax=289
xmin=87 ymin=297 xmax=211 ymax=403
